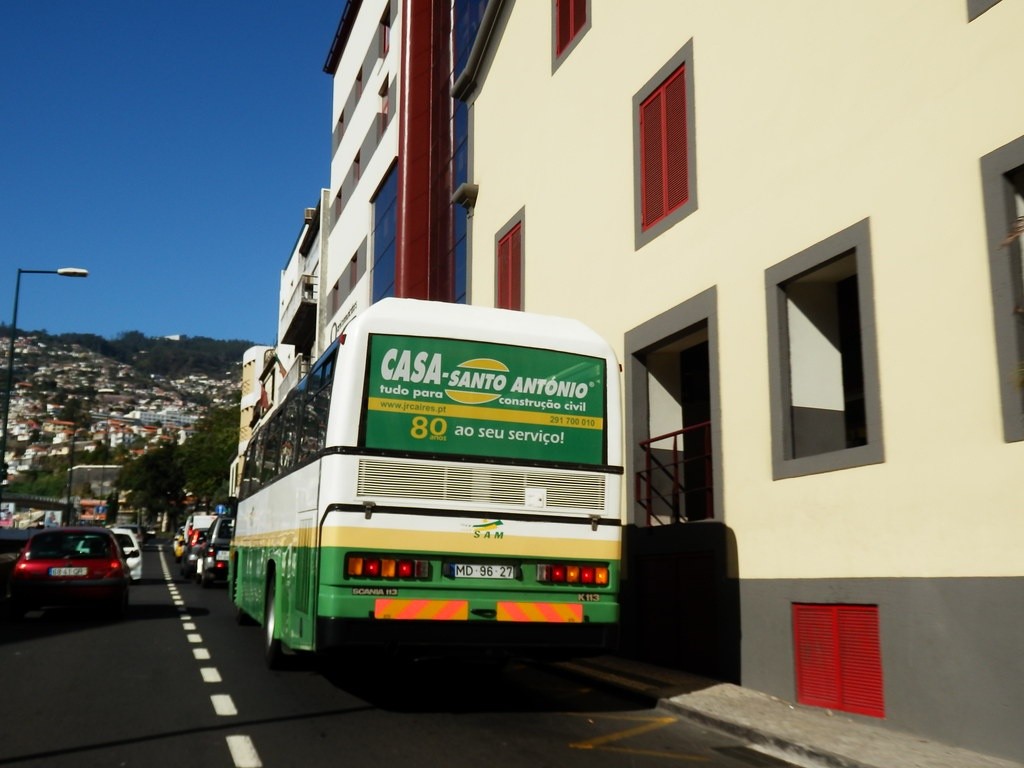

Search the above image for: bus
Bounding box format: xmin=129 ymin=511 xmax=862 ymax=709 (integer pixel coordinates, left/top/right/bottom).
xmin=225 ymin=296 xmax=624 ymax=672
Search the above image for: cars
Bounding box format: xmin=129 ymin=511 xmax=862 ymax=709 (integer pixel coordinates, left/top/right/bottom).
xmin=12 ymin=523 xmax=156 ymax=621
xmin=174 ymin=510 xmax=236 ymax=588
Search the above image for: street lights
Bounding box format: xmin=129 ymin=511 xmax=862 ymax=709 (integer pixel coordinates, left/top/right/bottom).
xmin=0 ymin=268 xmax=90 ymax=485
xmin=66 ymin=388 xmax=115 ymax=526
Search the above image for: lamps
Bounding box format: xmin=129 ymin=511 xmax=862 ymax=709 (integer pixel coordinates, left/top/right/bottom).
xmin=449 ymin=182 xmax=477 ymax=218
